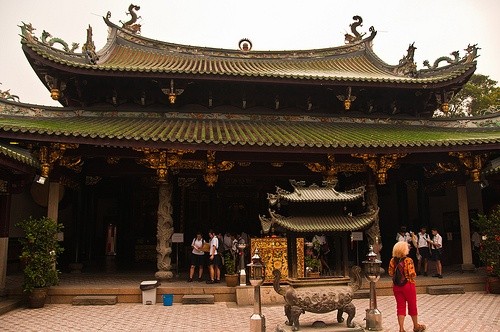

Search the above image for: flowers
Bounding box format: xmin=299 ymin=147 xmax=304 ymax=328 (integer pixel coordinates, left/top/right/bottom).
xmin=304 ymin=235 xmax=326 ymax=272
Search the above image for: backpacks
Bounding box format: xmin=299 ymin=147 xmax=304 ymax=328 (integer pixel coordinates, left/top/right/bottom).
xmin=393 ymin=256 xmax=408 ymax=286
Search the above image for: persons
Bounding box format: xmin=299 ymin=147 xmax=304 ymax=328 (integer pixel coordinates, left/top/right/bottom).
xmin=471 ymin=228 xmax=481 ymax=268
xmin=394 ymin=225 xmax=443 ymax=279
xmin=188 ymin=227 xmax=286 ymax=284
xmin=312 ymin=233 xmax=331 ymax=271
xmin=389 ymin=241 xmax=426 ymax=332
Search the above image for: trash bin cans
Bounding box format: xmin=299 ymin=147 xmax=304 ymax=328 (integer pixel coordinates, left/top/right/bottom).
xmin=140 ymin=280 xmax=158 ymax=304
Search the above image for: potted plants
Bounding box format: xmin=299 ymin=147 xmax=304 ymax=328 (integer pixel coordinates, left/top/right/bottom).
xmin=470 ymin=207 xmax=500 ymax=293
xmin=222 ymin=250 xmax=239 ymax=285
xmin=12 ymin=213 xmax=65 ymax=309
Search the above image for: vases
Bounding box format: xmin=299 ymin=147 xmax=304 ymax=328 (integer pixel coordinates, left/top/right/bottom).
xmin=309 ymin=270 xmax=320 ymax=278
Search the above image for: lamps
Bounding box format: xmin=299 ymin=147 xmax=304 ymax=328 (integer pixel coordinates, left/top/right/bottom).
xmin=361 ymin=246 xmax=384 ymax=277
xmin=34 ymin=175 xmax=47 ymax=185
xmin=248 ymin=248 xmax=268 ymax=286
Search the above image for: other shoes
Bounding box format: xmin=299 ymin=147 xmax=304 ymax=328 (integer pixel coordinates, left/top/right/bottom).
xmin=187 ymin=279 xmax=192 ymax=282
xmin=432 ymin=274 xmax=442 ymax=278
xmin=198 ymin=278 xmax=202 ymax=282
xmin=424 ymin=272 xmax=428 ymax=276
xmin=206 ymin=279 xmax=220 ymax=284
xmin=413 ymin=324 xmax=426 ymax=332
xmin=417 ymin=272 xmax=422 ymax=276
